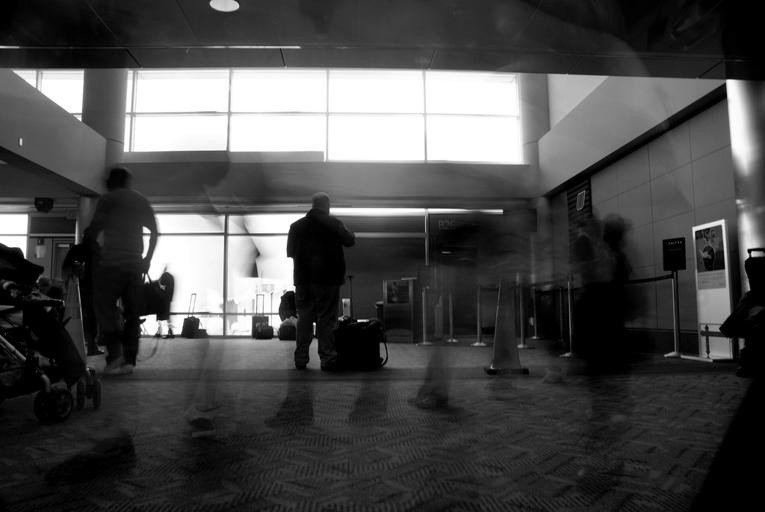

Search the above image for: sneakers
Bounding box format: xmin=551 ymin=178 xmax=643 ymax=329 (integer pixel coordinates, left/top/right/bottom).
xmin=105 ymin=357 xmax=132 ymax=375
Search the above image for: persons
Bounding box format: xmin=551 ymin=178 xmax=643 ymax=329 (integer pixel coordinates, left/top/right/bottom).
xmin=154 ymin=265 xmax=175 ymax=339
xmin=83 ymin=169 xmax=158 ymax=375
xmin=288 ymin=193 xmax=356 ymax=370
xmin=61 ymin=227 xmax=105 ymax=354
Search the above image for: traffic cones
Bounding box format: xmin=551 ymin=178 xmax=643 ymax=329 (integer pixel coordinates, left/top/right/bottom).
xmin=484 ymin=274 xmax=529 ymax=375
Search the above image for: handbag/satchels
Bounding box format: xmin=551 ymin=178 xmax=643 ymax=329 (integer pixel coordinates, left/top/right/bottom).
xmin=138 ymin=273 xmax=159 ymax=317
xmin=255 ymin=326 xmax=272 ymax=339
xmin=339 ymin=314 xmax=389 ymax=370
xmin=189 ymin=329 xmax=207 ymax=338
xmin=278 ymin=317 xmax=298 ymax=341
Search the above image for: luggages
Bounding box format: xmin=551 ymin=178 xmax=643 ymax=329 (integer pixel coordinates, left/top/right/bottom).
xmin=182 ymin=294 xmax=197 ymax=338
xmin=253 ymin=294 xmax=269 ymax=338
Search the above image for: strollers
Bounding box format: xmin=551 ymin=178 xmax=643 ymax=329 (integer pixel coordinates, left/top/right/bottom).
xmin=0 ymin=277 xmax=105 ymax=427
